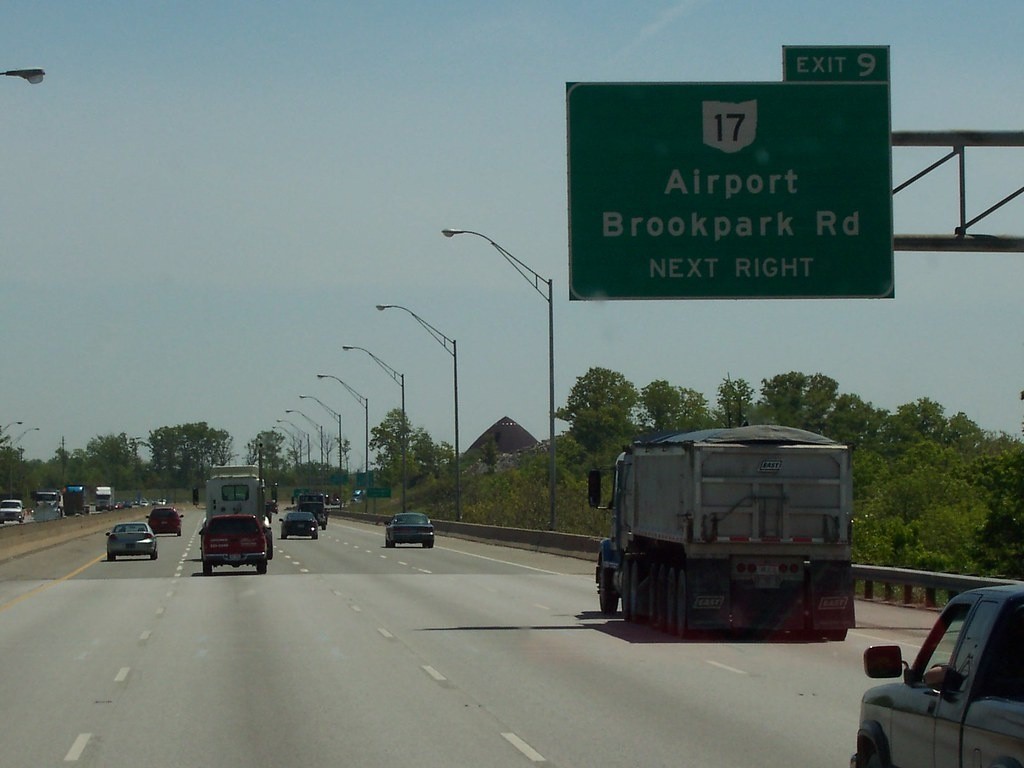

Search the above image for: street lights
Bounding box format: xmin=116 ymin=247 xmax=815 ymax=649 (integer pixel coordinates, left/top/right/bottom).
xmin=442 ymin=227 xmax=558 ymax=533
xmin=376 ymin=304 xmax=462 ymax=523
xmin=317 ymin=374 xmax=369 ymax=512
xmin=285 ymin=408 xmax=324 ymax=496
xmin=342 ymin=345 xmax=407 ymax=514
xmin=277 ymin=418 xmax=311 ymax=494
xmin=272 ymin=425 xmax=302 ymax=489
xmin=299 ymin=395 xmax=342 ymax=511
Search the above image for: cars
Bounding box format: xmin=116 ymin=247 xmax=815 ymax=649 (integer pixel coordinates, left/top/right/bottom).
xmin=297 ymin=491 xmax=341 ymax=530
xmin=279 ymin=511 xmax=319 ymax=540
xmin=197 ymin=514 xmax=269 ymax=577
xmin=0 ymin=499 xmax=25 ymax=524
xmin=115 ymin=495 xmax=167 ymax=510
xmin=384 ymin=512 xmax=436 ymax=548
xmin=105 ymin=522 xmax=159 ymax=561
xmin=146 ymin=505 xmax=184 ymax=536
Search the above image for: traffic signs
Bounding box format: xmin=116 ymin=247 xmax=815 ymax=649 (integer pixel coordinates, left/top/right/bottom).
xmin=564 ymin=42 xmax=896 ymax=303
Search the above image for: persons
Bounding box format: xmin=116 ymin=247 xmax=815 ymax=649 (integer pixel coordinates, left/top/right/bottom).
xmin=924 ymin=666 xmax=948 ymax=688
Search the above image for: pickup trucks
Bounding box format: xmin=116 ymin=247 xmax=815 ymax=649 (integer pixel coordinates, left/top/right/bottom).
xmin=849 ymin=584 xmax=1024 ymax=768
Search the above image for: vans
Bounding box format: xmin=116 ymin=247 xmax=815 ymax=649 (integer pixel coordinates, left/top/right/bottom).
xmin=204 ymin=465 xmax=272 ymax=559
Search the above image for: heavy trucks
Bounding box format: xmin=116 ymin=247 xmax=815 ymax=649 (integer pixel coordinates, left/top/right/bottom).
xmin=34 ymin=491 xmax=65 ymax=518
xmin=95 ymin=487 xmax=115 ymax=511
xmin=584 ymin=424 xmax=855 ymax=642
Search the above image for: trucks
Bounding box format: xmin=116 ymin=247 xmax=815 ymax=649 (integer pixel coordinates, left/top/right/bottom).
xmin=65 ymin=483 xmax=88 ymax=515
